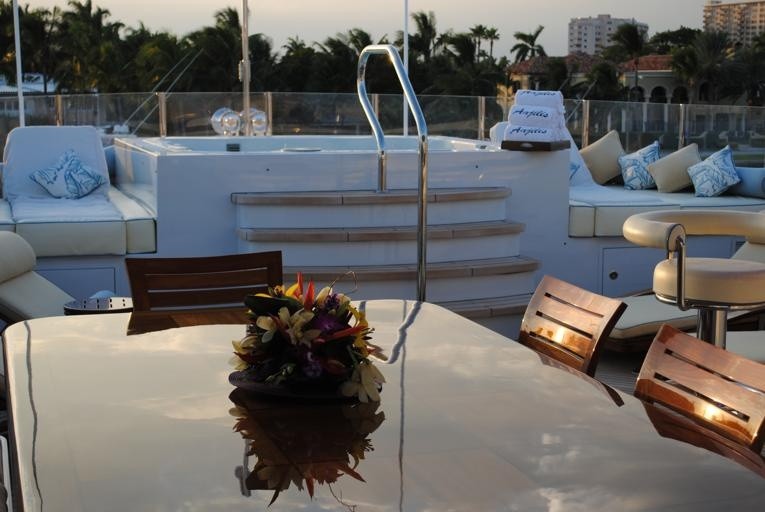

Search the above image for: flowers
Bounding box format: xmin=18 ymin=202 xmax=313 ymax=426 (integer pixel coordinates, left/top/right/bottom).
xmin=228 ymin=265 xmax=389 ymax=506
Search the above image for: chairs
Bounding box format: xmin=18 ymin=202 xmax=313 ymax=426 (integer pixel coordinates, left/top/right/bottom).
xmin=124 ymin=249 xmax=284 ymax=308
xmin=0 ymin=230 xmax=73 ymax=318
xmin=635 ymin=322 xmax=765 ymax=449
xmin=623 ymin=208 xmax=764 ymax=352
xmin=0 ymin=124 xmax=156 ymax=298
xmin=517 ymin=274 xmax=629 ymax=378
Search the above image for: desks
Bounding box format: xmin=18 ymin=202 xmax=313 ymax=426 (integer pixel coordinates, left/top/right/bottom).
xmin=2 ymin=300 xmax=764 ymax=512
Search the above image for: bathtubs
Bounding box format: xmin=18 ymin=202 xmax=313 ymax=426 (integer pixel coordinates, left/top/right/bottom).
xmin=113 ymin=134 xmax=570 ymax=302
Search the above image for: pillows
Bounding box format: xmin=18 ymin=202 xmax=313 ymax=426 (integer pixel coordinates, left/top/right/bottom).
xmin=31 ymin=150 xmax=107 ymax=198
xmin=578 ymin=130 xmax=741 ymax=197
